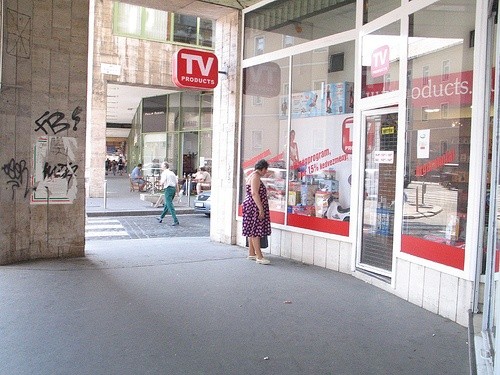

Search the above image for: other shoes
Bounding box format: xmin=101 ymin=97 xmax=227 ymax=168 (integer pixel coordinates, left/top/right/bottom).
xmin=172 ymin=221 xmax=179 ymax=225
xmin=248 ymin=254 xmax=256 ymax=259
xmin=255 ymin=258 xmax=270 ymax=264
xmin=156 ymin=217 xmax=162 ymax=222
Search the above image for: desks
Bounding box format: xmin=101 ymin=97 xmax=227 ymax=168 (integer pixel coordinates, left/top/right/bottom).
xmin=403 ymin=202 xmax=444 ymax=236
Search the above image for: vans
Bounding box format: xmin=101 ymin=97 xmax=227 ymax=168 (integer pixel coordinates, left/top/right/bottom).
xmin=244 ymin=166 xmax=287 ymax=193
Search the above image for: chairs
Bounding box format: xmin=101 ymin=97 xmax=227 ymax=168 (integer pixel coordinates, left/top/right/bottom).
xmin=128 ymin=172 xmax=141 ymax=192
xmin=191 ymin=173 xmax=208 ymax=196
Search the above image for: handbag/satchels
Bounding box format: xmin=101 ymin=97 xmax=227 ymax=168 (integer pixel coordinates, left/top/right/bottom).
xmin=246 ymin=234 xmax=268 ymax=247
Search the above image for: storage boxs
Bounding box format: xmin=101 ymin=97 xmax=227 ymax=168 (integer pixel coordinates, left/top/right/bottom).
xmin=289 ymin=190 xmax=301 ymax=206
xmin=279 ymin=88 xmax=321 ymax=119
xmin=363 ymin=199 xmax=378 ymax=227
xmin=287 ymin=205 xmax=293 ymax=214
xmin=321 ymin=80 xmax=354 ymax=116
xmin=319 ymin=169 xmax=336 ymax=180
xmin=289 ymin=181 xmax=303 ymax=191
xmin=302 ymin=174 xmax=318 ymax=183
xmin=377 ymin=207 xmax=394 ymax=237
xmin=315 ymin=179 xmax=339 ymax=194
xmin=300 ymin=184 xmax=321 ymax=206
xmin=242 ymin=167 xmax=287 ymax=213
xmin=364 ymin=168 xmax=379 ymax=197
xmin=291 ymin=205 xmax=316 ymax=217
xmin=315 ymin=190 xmax=339 ymax=219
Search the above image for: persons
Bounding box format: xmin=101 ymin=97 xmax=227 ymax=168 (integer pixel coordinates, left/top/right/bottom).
xmin=182 ymin=168 xmax=197 ymax=194
xmin=347 ymin=87 xmax=353 ymax=104
xmin=131 ymin=164 xmax=145 ymax=192
xmin=156 ymin=161 xmax=179 ymax=226
xmin=282 ymin=99 xmax=287 ymax=115
xmin=195 ymin=166 xmax=210 ymax=195
xmin=327 ymin=85 xmax=331 ymax=111
xmin=105 ymin=158 xmax=127 ymax=175
xmin=289 ymin=129 xmax=299 ymax=166
xmin=242 ymin=159 xmax=272 ymax=265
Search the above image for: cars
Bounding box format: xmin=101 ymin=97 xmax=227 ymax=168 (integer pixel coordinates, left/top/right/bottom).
xmin=194 ymin=191 xmax=210 ymax=217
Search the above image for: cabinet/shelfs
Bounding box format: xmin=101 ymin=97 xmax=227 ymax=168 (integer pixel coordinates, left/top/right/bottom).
xmin=450 ymin=135 xmax=493 ymax=219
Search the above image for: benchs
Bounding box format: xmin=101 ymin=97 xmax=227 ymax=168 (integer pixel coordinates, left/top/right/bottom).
xmin=140 ymin=194 xmax=181 ymax=207
